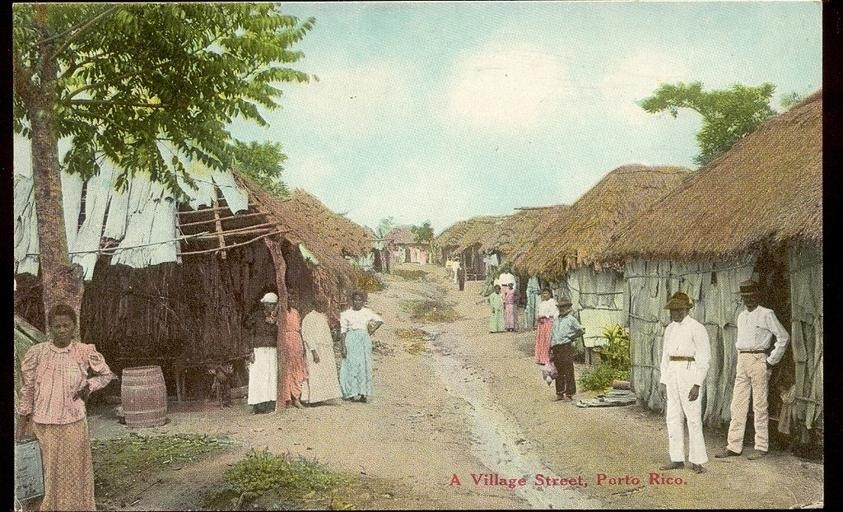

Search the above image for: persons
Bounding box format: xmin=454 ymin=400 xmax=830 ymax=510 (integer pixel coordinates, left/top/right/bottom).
xmin=339 ymin=290 xmax=384 ymax=403
xmin=483 ymin=249 xmax=517 ymax=333
xmin=534 ymin=288 xmax=559 ymax=365
xmin=244 ymin=292 xmax=282 ymax=414
xmin=16 ymin=304 xmax=113 ymax=512
xmin=300 ymin=295 xmax=344 ymax=407
xmin=270 ymin=288 xmax=311 ymax=412
xmin=446 ymin=255 xmax=466 ymax=291
xmin=356 ymin=244 xmax=444 ymax=275
xmin=715 ymin=280 xmax=792 ymax=460
xmin=545 ymin=296 xmax=586 ymax=401
xmin=659 ymin=290 xmax=712 ymax=473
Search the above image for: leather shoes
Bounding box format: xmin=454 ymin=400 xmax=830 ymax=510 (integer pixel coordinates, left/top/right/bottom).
xmin=747 ymin=450 xmax=767 ymax=460
xmin=716 ymin=448 xmax=741 ymax=457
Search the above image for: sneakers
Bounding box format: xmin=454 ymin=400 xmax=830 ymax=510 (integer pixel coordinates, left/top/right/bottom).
xmin=552 ymin=394 xmax=573 ymax=401
xmin=659 ymin=462 xmax=706 ymax=473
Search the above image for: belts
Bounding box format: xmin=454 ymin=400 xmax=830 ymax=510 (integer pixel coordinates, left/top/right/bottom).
xmin=669 ymin=356 xmax=694 ymax=361
xmin=740 ymin=350 xmax=765 ymax=353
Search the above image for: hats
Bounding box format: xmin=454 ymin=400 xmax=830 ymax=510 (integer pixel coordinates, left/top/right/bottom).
xmin=662 ymin=293 xmax=693 ymax=309
xmin=734 ymin=282 xmax=760 ymax=295
xmin=555 ymin=297 xmax=572 ymax=306
